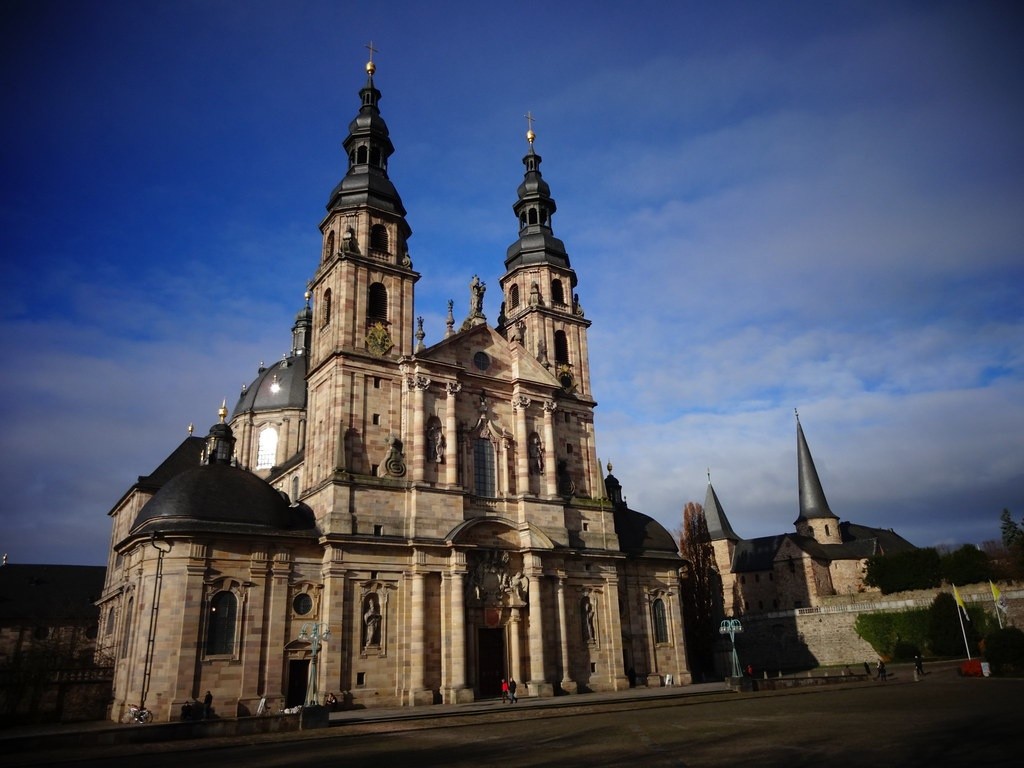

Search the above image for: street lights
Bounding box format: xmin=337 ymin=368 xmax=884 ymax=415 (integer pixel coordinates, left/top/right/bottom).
xmin=298 ymin=622 xmax=332 ymax=706
xmin=719 ymin=619 xmax=745 ymax=676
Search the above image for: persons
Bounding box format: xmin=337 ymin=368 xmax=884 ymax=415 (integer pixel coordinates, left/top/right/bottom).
xmin=463 ymin=549 xmax=528 ymax=606
xmin=582 ymin=602 xmax=595 ymax=640
xmin=529 ymin=436 xmax=544 ymax=475
xmin=426 ymin=420 xmax=446 ymax=464
xmin=325 ymin=692 xmax=338 ymax=712
xmin=180 ymin=701 xmax=194 ymax=722
xmin=363 ymin=598 xmax=382 ymax=645
xmin=200 ymin=690 xmax=214 ymax=721
xmin=508 ymin=677 xmax=519 ymax=704
xmin=502 ymin=679 xmax=512 ymax=703
xmin=842 ymin=653 xmax=926 ymax=682
xmin=471 ymin=275 xmax=483 ymax=314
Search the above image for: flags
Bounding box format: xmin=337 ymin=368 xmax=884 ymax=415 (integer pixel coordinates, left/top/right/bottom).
xmin=989 ymin=579 xmax=1009 ymax=615
xmin=952 ymin=582 xmax=970 ymax=622
